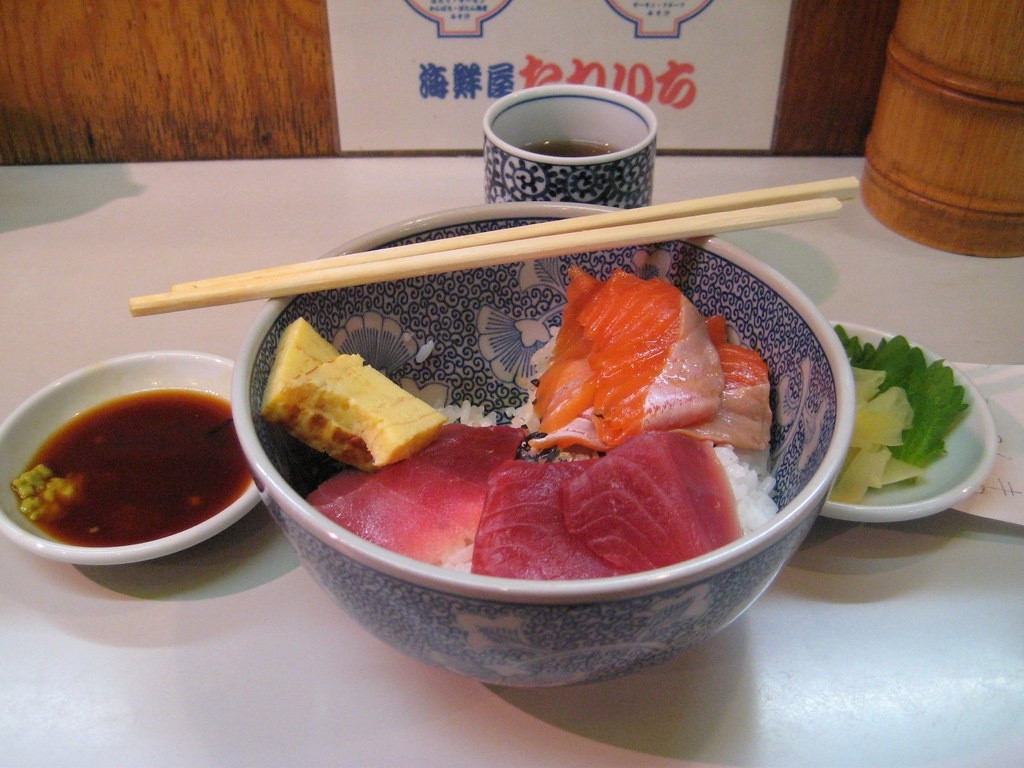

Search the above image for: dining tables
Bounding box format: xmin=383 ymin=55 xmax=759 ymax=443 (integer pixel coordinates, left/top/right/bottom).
xmin=1 ymin=151 xmax=1022 ymax=768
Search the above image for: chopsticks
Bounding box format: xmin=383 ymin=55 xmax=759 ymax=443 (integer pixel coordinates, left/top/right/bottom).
xmin=127 ymin=174 xmax=862 ymax=319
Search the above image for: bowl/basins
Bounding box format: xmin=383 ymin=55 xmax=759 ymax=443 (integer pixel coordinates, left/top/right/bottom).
xmin=232 ymin=202 xmax=855 ymax=694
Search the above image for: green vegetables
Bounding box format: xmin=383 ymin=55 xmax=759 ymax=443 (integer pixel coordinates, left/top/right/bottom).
xmin=834 ymin=324 xmax=968 ymax=505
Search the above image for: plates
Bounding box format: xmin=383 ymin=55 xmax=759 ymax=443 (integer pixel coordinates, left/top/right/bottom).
xmin=0 ymin=349 xmax=270 ymax=570
xmin=816 ymin=322 xmax=996 ymax=524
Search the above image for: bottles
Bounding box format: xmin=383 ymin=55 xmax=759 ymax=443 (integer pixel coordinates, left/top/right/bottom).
xmin=858 ymin=2 xmax=1023 ymax=264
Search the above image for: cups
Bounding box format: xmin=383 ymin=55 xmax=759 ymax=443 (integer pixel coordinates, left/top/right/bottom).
xmin=480 ymin=85 xmax=659 ymax=218
